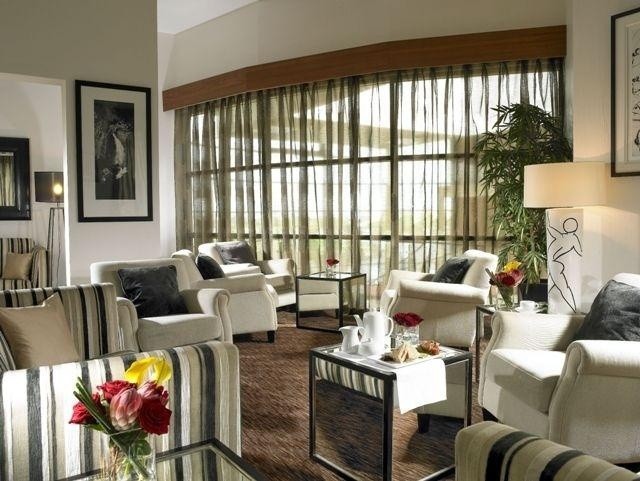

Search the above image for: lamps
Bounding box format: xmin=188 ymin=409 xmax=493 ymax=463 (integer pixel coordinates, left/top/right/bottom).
xmin=523 ymin=161 xmax=606 ymax=314
xmin=34 ymin=172 xmax=64 ymax=286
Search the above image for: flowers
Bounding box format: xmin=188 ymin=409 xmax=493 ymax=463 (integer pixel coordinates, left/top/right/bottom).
xmin=485 ymin=261 xmax=523 ymax=308
xmin=327 ymin=259 xmax=338 ymax=265
xmin=393 ymin=312 xmax=423 ymax=327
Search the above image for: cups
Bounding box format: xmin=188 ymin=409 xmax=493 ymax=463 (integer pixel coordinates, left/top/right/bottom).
xmin=325 ymin=264 xmax=336 ymax=275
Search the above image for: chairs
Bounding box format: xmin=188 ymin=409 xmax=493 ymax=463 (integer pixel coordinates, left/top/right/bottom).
xmin=380 ymin=249 xmax=498 ymax=348
xmin=478 ymin=273 xmax=640 ymax=465
xmin=454 ymin=421 xmax=640 ymax=481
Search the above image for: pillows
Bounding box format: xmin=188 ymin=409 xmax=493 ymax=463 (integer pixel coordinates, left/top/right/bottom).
xmin=431 ymin=257 xmax=470 ymax=283
xmin=572 ymin=280 xmax=640 ymax=346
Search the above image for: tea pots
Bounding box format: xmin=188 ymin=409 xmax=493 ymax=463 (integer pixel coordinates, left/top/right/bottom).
xmin=338 ymin=308 xmax=394 ymax=356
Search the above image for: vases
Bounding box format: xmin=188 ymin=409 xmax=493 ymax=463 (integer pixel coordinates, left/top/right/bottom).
xmin=327 ymin=266 xmax=336 ymax=276
xmin=490 ymin=285 xmax=520 ymax=311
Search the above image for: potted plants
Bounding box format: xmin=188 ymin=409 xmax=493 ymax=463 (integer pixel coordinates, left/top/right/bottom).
xmin=472 ymin=102 xmax=573 ymax=300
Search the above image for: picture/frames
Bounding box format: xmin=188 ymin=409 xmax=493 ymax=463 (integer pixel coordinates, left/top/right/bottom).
xmin=75 ymin=80 xmax=153 ymax=223
xmin=0 ymin=137 xmax=31 ymax=220
xmin=611 ymin=8 xmax=640 ymax=177
